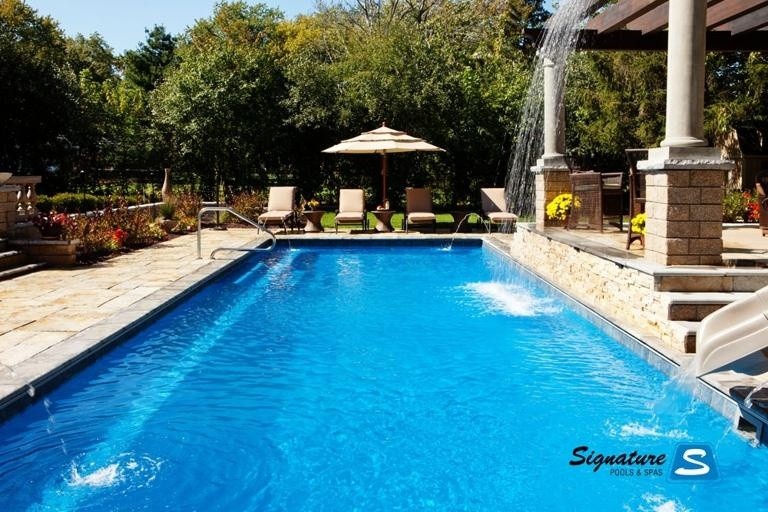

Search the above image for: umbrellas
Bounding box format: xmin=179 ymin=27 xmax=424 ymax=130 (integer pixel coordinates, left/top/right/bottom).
xmin=319 ymin=120 xmax=447 ymax=210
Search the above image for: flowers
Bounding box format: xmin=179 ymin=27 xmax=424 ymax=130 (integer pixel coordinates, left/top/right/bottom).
xmin=739 ymin=189 xmax=761 ymax=222
xmin=546 ymin=193 xmax=585 ymax=221
xmin=630 ymin=211 xmax=647 ymax=236
xmin=33 ymin=209 xmax=70 ymax=236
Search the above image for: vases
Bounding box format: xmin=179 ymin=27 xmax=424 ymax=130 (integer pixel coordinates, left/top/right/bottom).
xmin=39 ymin=234 xmax=62 ymax=240
xmin=559 ymin=211 xmax=582 ymax=229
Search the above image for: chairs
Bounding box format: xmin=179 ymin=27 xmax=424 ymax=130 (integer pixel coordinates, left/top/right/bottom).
xmin=566 ymin=170 xmax=625 ymax=233
xmin=255 ymin=186 xmax=520 ymax=235
xmin=624 ymin=148 xmax=649 ymax=248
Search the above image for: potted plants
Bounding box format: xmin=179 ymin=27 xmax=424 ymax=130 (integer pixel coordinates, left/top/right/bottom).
xmin=215 ymin=211 xmax=232 ymax=230
xmin=158 ymin=202 xmax=177 ymax=231
